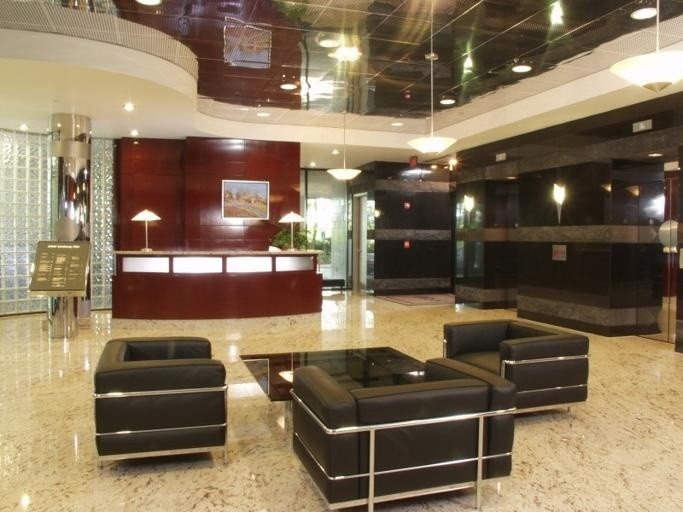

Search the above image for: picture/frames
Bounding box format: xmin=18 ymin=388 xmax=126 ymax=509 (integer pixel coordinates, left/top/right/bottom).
xmin=221 ymin=180 xmax=270 ymax=220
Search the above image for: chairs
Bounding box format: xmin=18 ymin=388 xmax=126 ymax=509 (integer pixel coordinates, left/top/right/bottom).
xmin=443 ymin=318 xmax=589 ymax=415
xmin=289 ymin=357 xmax=517 ymax=512
xmin=92 ymin=336 xmax=228 ymax=468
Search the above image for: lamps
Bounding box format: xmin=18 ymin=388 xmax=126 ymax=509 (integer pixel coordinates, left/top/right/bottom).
xmin=131 ymin=209 xmax=162 ymax=252
xmin=278 ymin=210 xmax=306 ymax=252
xmin=408 ymin=0 xmax=458 ymax=160
xmin=326 ymin=56 xmax=362 ymax=180
xmin=611 ymin=0 xmax=683 ymax=94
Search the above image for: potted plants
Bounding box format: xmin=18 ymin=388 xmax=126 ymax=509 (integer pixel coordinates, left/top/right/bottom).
xmin=271 ymin=224 xmax=311 ymax=250
